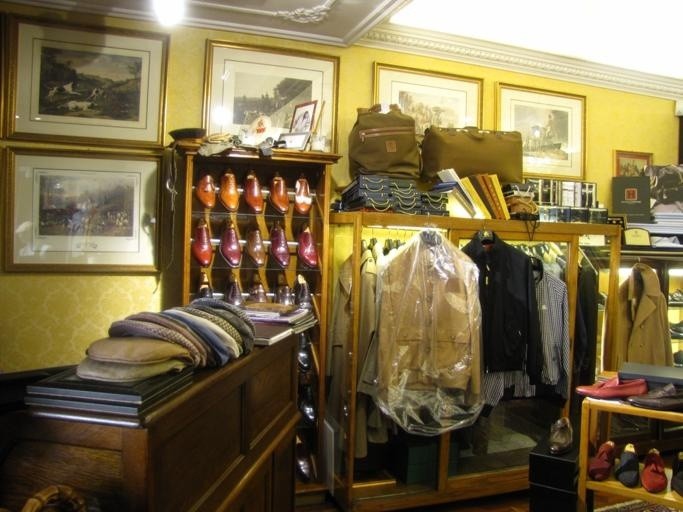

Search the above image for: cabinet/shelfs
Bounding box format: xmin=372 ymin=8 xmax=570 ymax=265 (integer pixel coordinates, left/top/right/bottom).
xmin=540 ymin=206 xmax=683 ymax=382
xmin=326 ymin=211 xmax=618 ymax=512
xmin=172 ymin=141 xmax=345 ymax=512
xmin=0 ymin=332 xmax=299 ymax=512
xmin=578 ymin=394 xmax=683 ymax=512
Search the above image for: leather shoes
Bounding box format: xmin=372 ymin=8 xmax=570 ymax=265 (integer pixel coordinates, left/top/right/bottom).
xmin=641 ymin=448 xmax=669 ymax=493
xmin=270 ymin=220 xmax=290 ymax=268
xmin=268 ymin=172 xmax=289 ymax=214
xmin=273 ymin=271 xmax=294 ymax=305
xmin=671 ymin=452 xmax=683 ymax=496
xmin=244 ymin=170 xmax=264 ymax=213
xmin=298 ymin=222 xmax=318 ymax=268
xmin=297 ymin=331 xmax=313 ymax=372
xmin=197 ymin=273 xmax=213 ymax=299
xmin=628 ymin=382 xmax=683 ymax=412
xmin=575 ymin=377 xmax=648 ymax=399
xmin=194 ymin=168 xmax=216 ymax=209
xmin=225 ymin=273 xmax=244 ymax=307
xmin=298 ymin=371 xmax=317 ymax=424
xmin=192 ymin=216 xmax=213 ymax=268
xmin=294 ymin=273 xmax=314 ymax=309
xmin=589 ymin=440 xmax=615 ymax=481
xmin=294 ymin=173 xmax=312 ymax=214
xmin=615 ymin=443 xmax=639 ymax=487
xmin=218 ymin=168 xmax=240 ymax=212
xmin=295 ymin=428 xmax=318 ymax=483
xmin=248 ymin=270 xmax=269 ymax=302
xmin=219 ymin=218 xmax=241 ymax=268
xmin=245 ymin=220 xmax=266 ymax=267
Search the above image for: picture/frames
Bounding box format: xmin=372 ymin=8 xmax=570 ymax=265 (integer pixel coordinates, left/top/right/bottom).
xmin=613 ymin=148 xmax=654 ymax=177
xmin=371 ymin=61 xmax=484 ymax=130
xmin=0 ymin=13 xmax=171 ymax=149
xmin=493 ymin=81 xmax=587 ymax=182
xmin=0 ymin=145 xmax=163 ymax=273
xmin=201 ymin=37 xmax=340 ymax=154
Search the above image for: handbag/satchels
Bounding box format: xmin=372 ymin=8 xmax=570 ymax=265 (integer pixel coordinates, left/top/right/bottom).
xmin=348 ymin=104 xmax=419 ymax=179
xmin=421 ymin=126 xmax=524 ymax=184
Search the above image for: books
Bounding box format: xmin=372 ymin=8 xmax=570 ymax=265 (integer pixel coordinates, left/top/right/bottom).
xmin=242 ymin=303 xmax=317 ymax=345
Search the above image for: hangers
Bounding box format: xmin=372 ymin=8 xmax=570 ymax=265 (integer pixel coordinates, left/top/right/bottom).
xmin=477 ymin=227 xmax=494 ymax=250
xmin=383 ymin=238 xmax=392 ymax=257
xmin=512 ymin=243 xmax=518 ymax=252
xmin=394 ymin=239 xmax=404 ymax=250
xmin=359 ymin=239 xmax=367 ymax=257
xmin=370 ymin=237 xmax=376 ymax=250
xmin=525 ymin=244 xmax=531 ymax=254
xmin=519 ymin=242 xmax=524 ymax=257
xmin=536 ymin=242 xmax=541 ymax=255
xmin=421 ymin=229 xmax=443 ymax=246
xmin=530 ymin=244 xmax=537 ymax=254
xmin=544 ymin=242 xmax=552 ymax=252
xmin=632 ymin=253 xmax=643 ymax=283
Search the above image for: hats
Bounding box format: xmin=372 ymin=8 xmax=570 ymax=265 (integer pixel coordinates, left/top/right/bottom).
xmin=198 ymin=133 xmax=233 ymax=156
xmin=169 ymin=128 xmax=206 ymax=151
xmin=73 ymin=298 xmax=255 ymax=382
